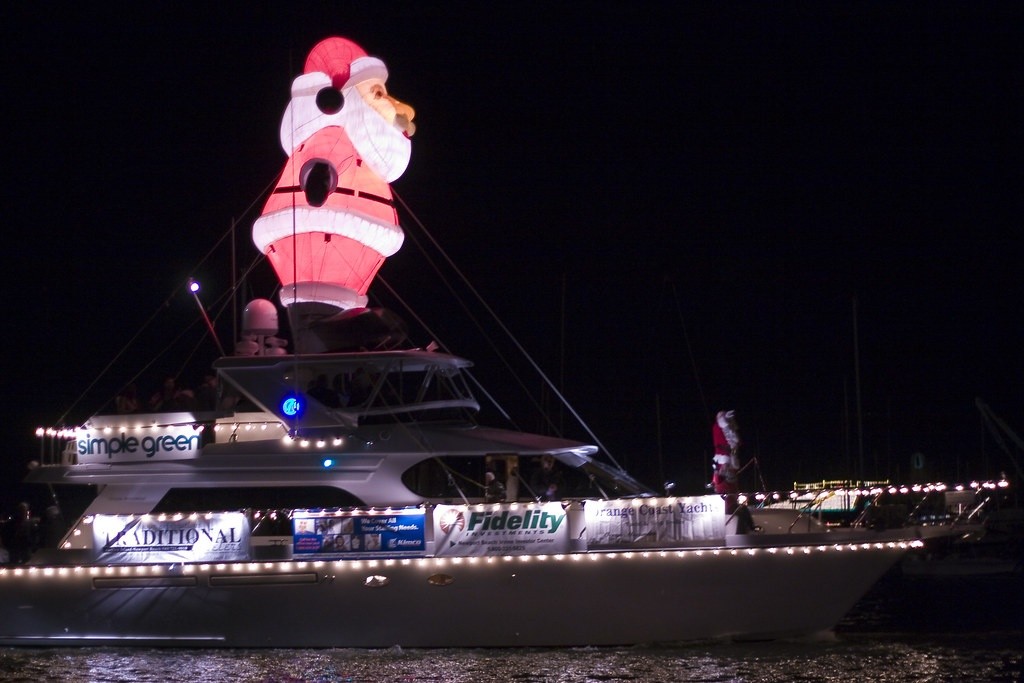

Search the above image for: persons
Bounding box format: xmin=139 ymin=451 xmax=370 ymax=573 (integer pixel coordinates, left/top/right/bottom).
xmin=485 ymin=471 xmax=503 ymax=500
xmin=115 ymin=380 xmax=136 ymax=413
xmin=197 ymin=374 xmax=218 ymax=411
xmin=711 ymin=410 xmax=743 ymax=494
xmin=301 ymin=371 xmax=335 ymax=409
xmin=148 ymin=378 xmax=179 ymax=411
xmin=537 ymin=454 xmax=563 ymax=499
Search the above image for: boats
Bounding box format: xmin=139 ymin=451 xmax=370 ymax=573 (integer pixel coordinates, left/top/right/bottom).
xmin=0 ymin=33 xmax=1009 ymax=652
xmin=898 ymin=393 xmax=1023 ymax=584
